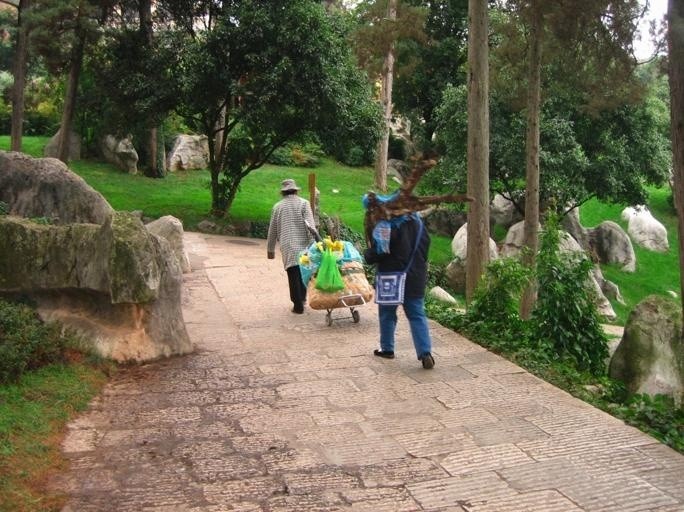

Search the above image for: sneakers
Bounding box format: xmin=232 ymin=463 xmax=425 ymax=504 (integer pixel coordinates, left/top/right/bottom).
xmin=373 ymin=349 xmax=396 ymax=359
xmin=420 ymin=352 xmax=434 ymax=368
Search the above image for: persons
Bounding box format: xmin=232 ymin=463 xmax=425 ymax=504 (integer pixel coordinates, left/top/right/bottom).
xmin=363 ymin=188 xmax=436 ymax=368
xmin=266 ymin=178 xmax=326 ymax=316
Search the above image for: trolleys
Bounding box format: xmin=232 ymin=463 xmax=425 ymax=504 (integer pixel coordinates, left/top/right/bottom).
xmin=325 ymin=294 xmax=366 ymax=326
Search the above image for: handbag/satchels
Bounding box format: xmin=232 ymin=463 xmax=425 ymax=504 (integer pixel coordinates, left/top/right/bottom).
xmin=313 ymin=251 xmax=344 ymax=293
xmin=374 ymin=271 xmax=406 ymax=306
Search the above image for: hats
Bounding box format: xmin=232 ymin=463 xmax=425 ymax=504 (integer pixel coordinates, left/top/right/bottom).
xmin=279 ymin=179 xmax=299 ymax=192
